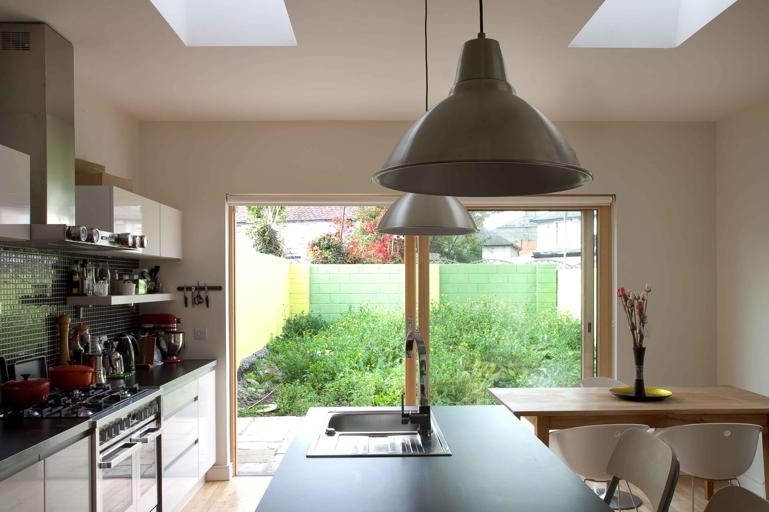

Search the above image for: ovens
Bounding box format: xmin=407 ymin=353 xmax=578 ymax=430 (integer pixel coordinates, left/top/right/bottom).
xmin=96 ymin=419 xmax=160 ymax=512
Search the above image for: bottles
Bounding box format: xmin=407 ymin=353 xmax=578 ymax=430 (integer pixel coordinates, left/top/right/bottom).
xmin=70 ymin=331 xmax=106 ymax=384
xmin=71 ymin=260 xmax=80 ymax=293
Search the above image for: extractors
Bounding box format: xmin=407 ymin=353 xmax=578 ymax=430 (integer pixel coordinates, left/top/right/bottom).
xmin=0 ymin=20 xmax=149 ymax=255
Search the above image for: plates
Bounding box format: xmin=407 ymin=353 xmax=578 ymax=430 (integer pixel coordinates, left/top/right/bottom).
xmin=609 ymin=385 xmax=672 ymax=401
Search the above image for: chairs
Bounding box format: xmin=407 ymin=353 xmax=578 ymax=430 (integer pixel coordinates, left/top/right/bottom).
xmin=602 ymin=428 xmax=681 ymax=511
xmin=656 ymin=423 xmax=762 ymax=511
xmin=547 ymin=426 xmax=652 ymax=511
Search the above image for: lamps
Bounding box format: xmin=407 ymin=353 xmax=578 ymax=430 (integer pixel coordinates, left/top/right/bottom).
xmin=370 ymin=0 xmax=592 ymax=196
xmin=375 ymin=2 xmax=479 ymax=237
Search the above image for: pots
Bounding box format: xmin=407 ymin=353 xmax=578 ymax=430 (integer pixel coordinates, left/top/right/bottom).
xmin=3 ymin=372 xmax=49 ymax=403
xmin=47 ymin=360 xmax=95 ymax=387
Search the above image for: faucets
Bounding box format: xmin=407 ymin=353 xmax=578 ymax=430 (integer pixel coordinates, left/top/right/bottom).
xmin=400 ymin=329 xmax=431 ymax=435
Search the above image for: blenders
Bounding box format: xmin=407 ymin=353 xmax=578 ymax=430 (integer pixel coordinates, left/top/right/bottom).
xmin=140 ymin=313 xmax=185 ymax=362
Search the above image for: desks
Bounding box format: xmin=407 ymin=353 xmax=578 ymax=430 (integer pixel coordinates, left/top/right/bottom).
xmin=486 ymin=385 xmax=763 ymax=497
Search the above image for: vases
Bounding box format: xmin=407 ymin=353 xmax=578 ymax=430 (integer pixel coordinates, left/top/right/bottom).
xmin=633 ymin=350 xmax=648 ymax=401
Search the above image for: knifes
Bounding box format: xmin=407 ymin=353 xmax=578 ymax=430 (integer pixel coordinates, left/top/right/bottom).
xmin=183 ymin=284 xmax=187 ymax=307
xmin=204 ymin=283 xmax=209 ymax=308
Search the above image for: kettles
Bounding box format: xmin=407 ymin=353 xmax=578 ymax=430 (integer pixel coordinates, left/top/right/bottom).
xmin=111 ymin=332 xmax=142 ymax=371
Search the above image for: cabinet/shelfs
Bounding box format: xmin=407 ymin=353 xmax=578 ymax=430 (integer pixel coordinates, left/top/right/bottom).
xmin=1 ymin=147 xmax=30 ymax=241
xmin=192 ymin=369 xmax=218 ymax=480
xmin=0 ymin=433 xmax=93 ymax=511
xmin=77 ymin=185 xmax=182 ymax=257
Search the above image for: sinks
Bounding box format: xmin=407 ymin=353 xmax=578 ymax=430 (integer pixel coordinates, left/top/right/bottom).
xmin=322 ymin=407 xmax=439 ymax=434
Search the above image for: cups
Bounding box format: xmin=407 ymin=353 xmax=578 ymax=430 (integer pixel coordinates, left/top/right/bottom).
xmin=93 ymin=283 xmax=109 ymax=296
xmin=120 ymin=283 xmax=136 ymax=295
xmin=105 ymin=350 xmax=125 ymax=375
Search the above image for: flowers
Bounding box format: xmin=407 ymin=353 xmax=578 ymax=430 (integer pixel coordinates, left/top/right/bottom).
xmin=615 ymin=287 xmax=649 ymax=347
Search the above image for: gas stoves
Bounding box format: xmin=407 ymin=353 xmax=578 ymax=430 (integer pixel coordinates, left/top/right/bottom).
xmin=0 ymin=383 xmax=160 ymax=452
